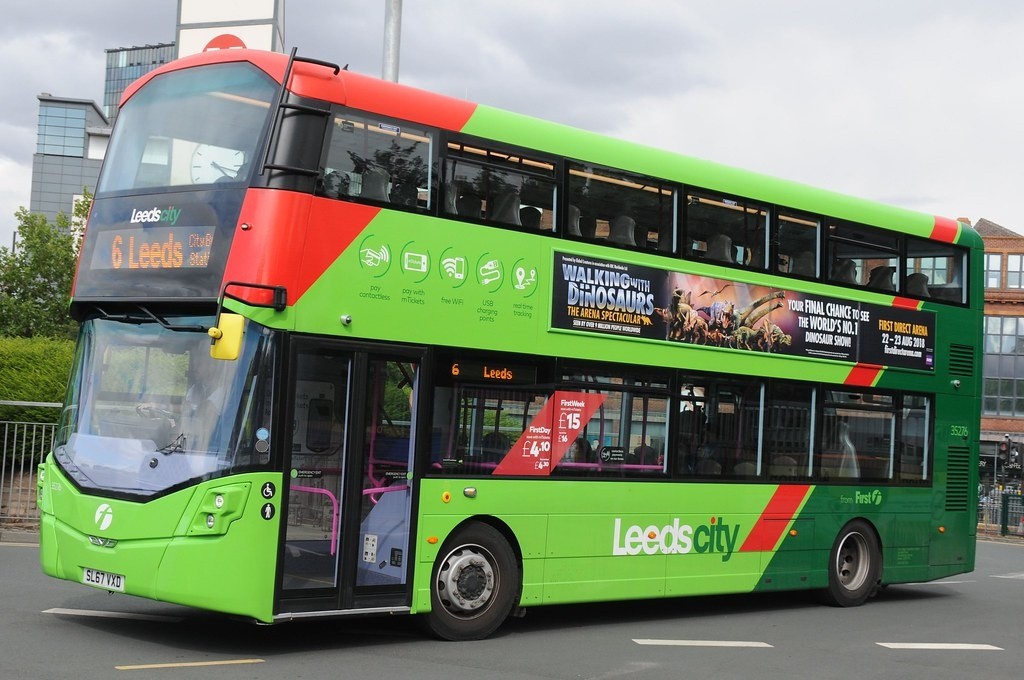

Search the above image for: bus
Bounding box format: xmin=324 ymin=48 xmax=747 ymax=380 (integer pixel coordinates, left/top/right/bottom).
xmin=35 ymin=46 xmax=985 ymax=642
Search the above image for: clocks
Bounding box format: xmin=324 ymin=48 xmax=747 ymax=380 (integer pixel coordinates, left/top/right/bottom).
xmin=190 ymin=142 xmax=244 ymax=184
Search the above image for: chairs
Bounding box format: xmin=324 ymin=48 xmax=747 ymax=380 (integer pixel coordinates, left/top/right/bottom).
xmin=607 ymin=215 xmax=636 ymax=246
xmin=829 ymin=258 xmax=857 ymax=285
xmin=704 ymin=235 xmax=734 ymax=264
xmin=579 ymin=217 xmax=597 ymax=238
xmin=866 ymin=266 xmax=896 ymax=292
xmin=769 ymin=457 xmax=797 ymax=476
xmin=696 ymin=460 xmax=720 ymax=478
xmin=633 ymin=227 xmax=648 ymax=248
xmin=481 ymin=432 xmax=693 ymax=477
xmin=360 ymin=168 xmax=389 ymax=202
xmin=568 ymin=204 xmax=581 ymax=236
xmin=907 ymin=273 xmax=930 ymax=297
xmin=790 ymin=251 xmax=815 ymax=277
xmin=324 ymin=171 xmax=349 ymax=194
xmin=392 ymin=185 xmax=419 ymax=209
xmin=456 ymin=196 xmax=483 ymax=217
xmin=438 ymin=182 xmax=457 ymax=215
xmin=734 ymin=462 xmax=757 ymax=475
xmin=519 ymin=207 xmax=542 ymax=230
xmin=489 ymin=191 xmax=522 ymax=226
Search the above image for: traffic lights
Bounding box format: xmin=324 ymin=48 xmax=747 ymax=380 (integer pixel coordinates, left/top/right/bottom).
xmin=998 ymin=441 xmax=1010 ymax=463
